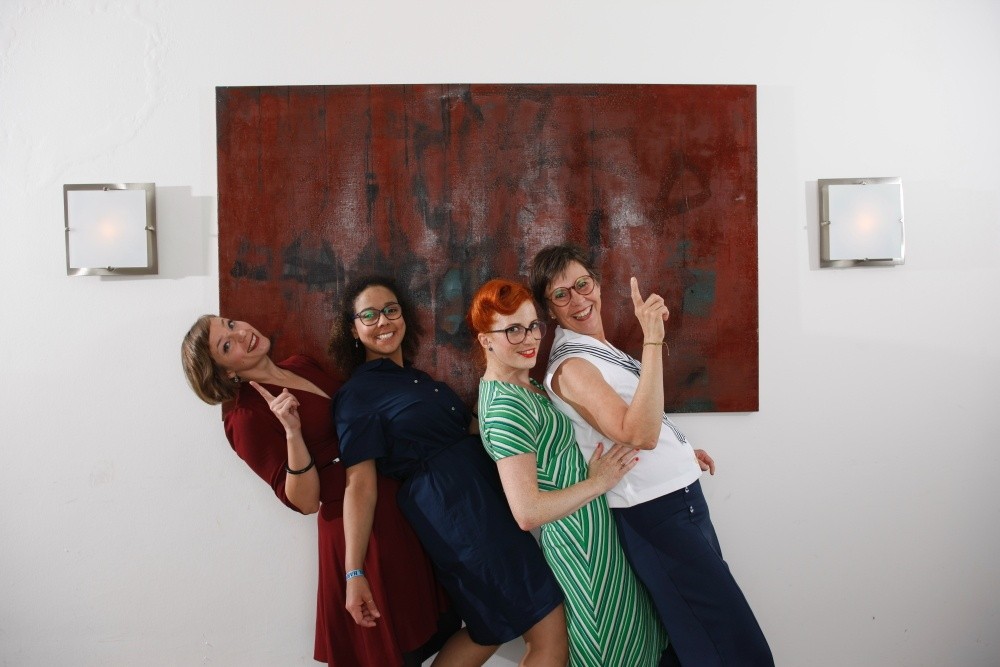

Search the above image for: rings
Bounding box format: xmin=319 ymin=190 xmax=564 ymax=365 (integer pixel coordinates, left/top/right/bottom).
xmin=619 ymin=459 xmax=625 ymax=466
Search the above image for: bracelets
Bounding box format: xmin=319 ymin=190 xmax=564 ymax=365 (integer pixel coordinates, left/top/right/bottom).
xmin=643 ymin=342 xmax=665 ymax=345
xmin=346 ymin=569 xmax=364 ymax=580
xmin=284 ymin=455 xmax=314 ymax=475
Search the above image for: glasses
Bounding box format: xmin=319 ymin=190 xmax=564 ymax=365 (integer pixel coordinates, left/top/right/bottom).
xmin=483 ymin=320 xmax=547 ymax=345
xmin=545 ymin=272 xmax=595 ymax=307
xmin=352 ymin=302 xmax=402 ymax=326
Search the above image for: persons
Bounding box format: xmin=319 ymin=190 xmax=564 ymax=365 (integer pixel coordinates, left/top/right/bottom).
xmin=327 ymin=276 xmax=568 ymax=667
xmin=180 ymin=315 xmax=443 ymax=667
xmin=469 ymin=277 xmax=668 ymax=667
xmin=530 ymin=244 xmax=776 ymax=667
